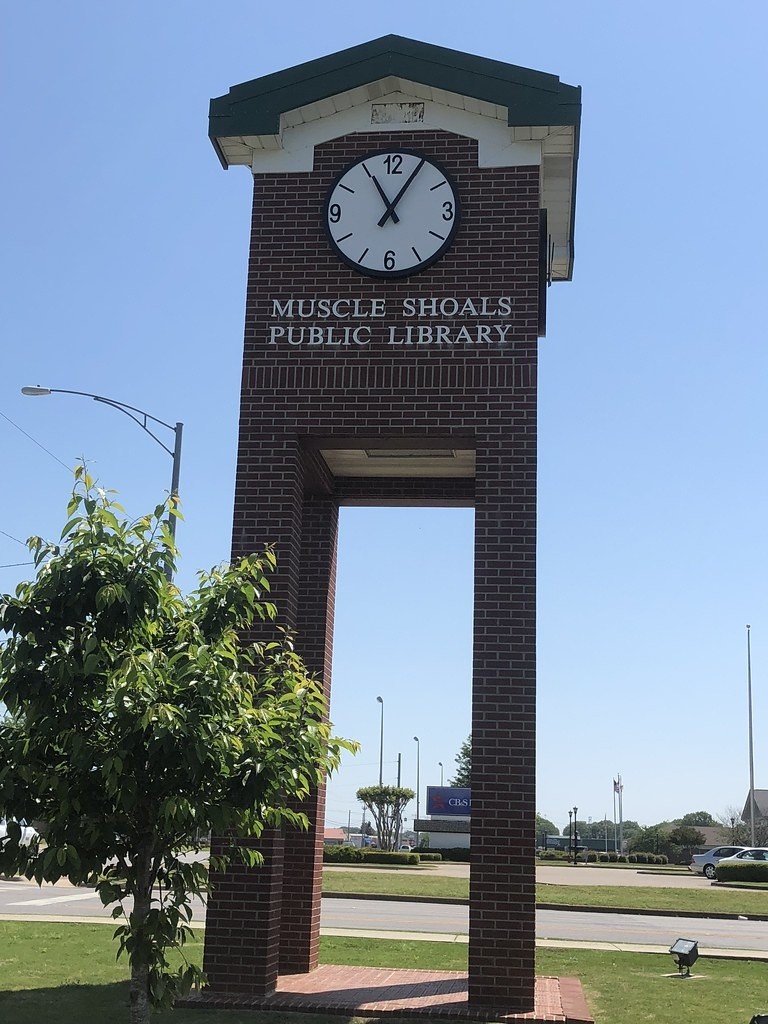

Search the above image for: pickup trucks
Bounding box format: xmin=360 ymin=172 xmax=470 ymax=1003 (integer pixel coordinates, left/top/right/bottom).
xmin=400 ymin=845 xmax=411 ymax=852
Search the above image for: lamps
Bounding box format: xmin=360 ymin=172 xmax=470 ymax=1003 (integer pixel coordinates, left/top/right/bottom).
xmin=670 ymin=938 xmax=701 ymax=977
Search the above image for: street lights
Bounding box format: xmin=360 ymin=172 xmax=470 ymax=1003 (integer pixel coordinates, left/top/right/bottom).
xmin=656 ymin=832 xmax=658 ymax=855
xmin=17 ymin=384 xmax=183 ymax=942
xmin=568 ymin=811 xmax=573 ymax=863
xmin=438 ymin=762 xmax=443 ymax=787
xmin=413 ymin=737 xmax=419 ymax=848
xmin=730 ymin=817 xmax=736 ymax=845
xmin=573 ymin=806 xmax=578 ymax=865
xmin=376 ymin=694 xmax=384 ymax=851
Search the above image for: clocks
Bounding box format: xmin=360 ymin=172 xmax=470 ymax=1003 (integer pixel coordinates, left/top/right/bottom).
xmin=323 ymin=149 xmax=460 ymax=277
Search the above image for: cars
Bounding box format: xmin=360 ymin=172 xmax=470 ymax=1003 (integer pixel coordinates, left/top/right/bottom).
xmin=0 ymin=814 xmax=40 ymax=848
xmin=718 ymin=847 xmax=768 ymax=864
xmin=341 ymin=842 xmax=355 ymax=847
xmin=690 ymin=846 xmax=752 ymax=880
